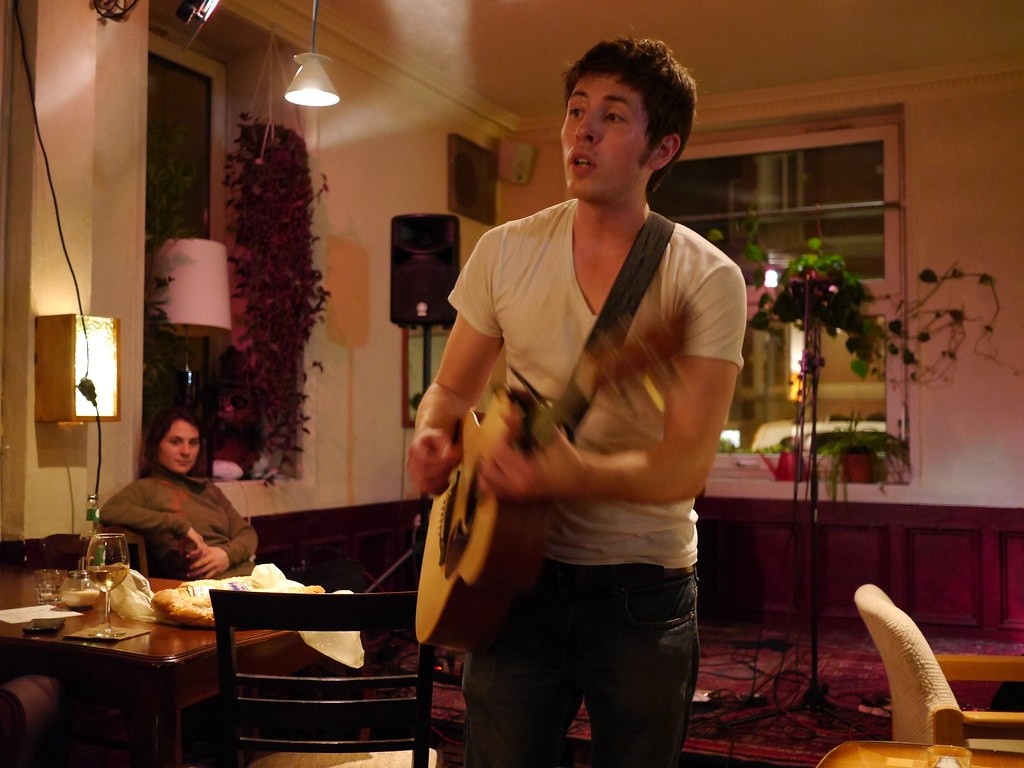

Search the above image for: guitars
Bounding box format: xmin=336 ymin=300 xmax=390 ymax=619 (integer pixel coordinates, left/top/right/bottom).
xmin=415 ymin=314 xmax=684 ymax=651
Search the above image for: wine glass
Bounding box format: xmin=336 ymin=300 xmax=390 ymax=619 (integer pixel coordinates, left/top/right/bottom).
xmin=85 ymin=533 xmax=130 ymax=637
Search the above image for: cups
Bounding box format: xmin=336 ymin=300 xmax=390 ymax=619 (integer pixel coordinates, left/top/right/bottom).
xmin=34 ymin=569 xmax=68 ymax=604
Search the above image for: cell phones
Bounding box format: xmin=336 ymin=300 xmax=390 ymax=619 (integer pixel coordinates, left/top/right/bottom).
xmin=23 ymin=618 xmax=64 ymax=634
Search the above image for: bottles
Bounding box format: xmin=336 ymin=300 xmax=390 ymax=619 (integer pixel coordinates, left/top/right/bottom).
xmin=60 ymin=571 xmax=100 ymax=610
xmin=78 ymin=493 xmax=105 ymax=572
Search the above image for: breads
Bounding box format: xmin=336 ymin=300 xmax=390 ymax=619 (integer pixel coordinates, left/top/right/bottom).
xmin=150 ymin=588 xmax=218 ymax=628
xmin=285 ymin=585 xmax=326 ymax=594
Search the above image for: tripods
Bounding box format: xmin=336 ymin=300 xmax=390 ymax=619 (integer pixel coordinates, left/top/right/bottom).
xmin=715 ymin=275 xmax=884 ymax=744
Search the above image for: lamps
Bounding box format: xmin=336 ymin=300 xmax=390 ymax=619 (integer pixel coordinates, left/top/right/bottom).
xmin=284 ymin=0 xmax=341 ymax=106
xmin=34 ymin=313 xmax=122 ymax=421
xmin=151 ymin=237 xmax=232 ymax=409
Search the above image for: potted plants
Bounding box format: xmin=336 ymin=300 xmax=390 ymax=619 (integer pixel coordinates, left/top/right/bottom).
xmin=711 ymin=219 xmax=1011 ymax=386
xmin=815 ymin=412 xmax=912 ymax=505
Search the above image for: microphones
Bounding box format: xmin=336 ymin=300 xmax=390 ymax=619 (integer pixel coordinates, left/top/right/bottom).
xmin=819 ymin=284 xmax=839 ymax=308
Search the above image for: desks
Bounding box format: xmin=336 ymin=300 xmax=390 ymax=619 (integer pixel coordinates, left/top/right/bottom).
xmin=815 ymin=740 xmax=1024 ymax=768
xmin=0 ymin=564 xmax=366 ymax=768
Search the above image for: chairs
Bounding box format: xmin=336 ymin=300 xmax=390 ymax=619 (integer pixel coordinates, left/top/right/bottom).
xmin=855 ymin=584 xmax=1024 ymax=753
xmin=207 ymin=588 xmax=439 ymax=768
xmin=101 ymin=525 xmax=149 ymax=575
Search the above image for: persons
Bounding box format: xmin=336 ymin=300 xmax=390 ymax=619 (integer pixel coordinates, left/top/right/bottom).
xmin=99 ymin=405 xmax=258 ymax=581
xmin=406 ymin=37 xmax=747 ymax=768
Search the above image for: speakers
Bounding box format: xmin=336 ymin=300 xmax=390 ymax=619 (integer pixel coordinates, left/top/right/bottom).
xmin=391 ymin=214 xmax=461 ymax=328
